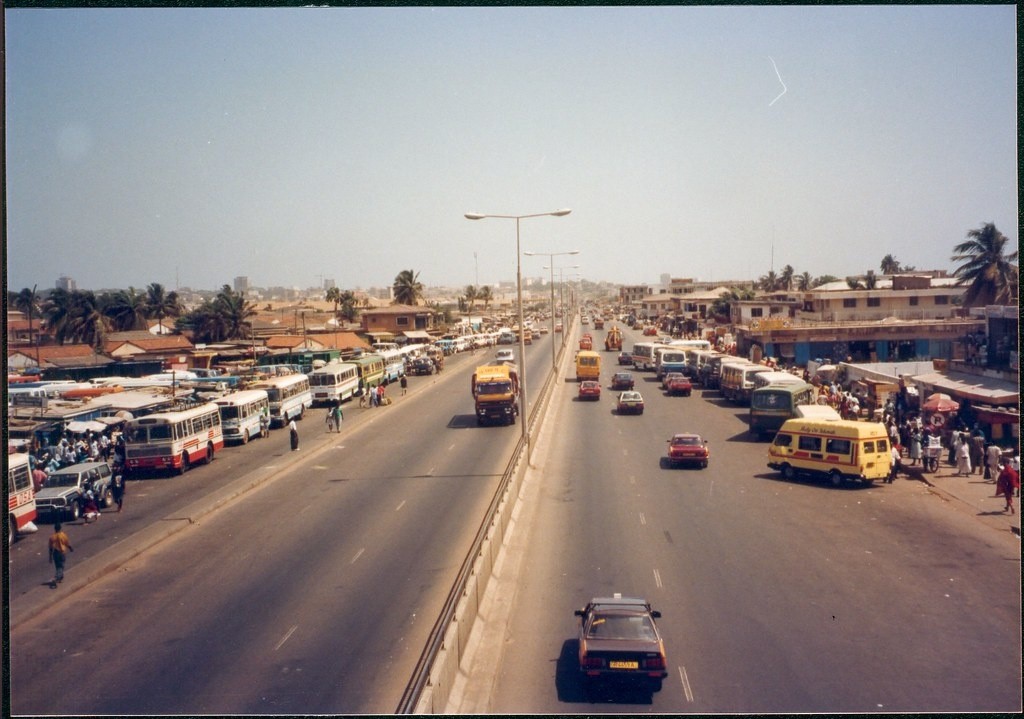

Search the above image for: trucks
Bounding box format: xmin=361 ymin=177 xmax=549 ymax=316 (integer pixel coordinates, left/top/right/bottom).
xmin=605 ymin=326 xmax=623 ymax=351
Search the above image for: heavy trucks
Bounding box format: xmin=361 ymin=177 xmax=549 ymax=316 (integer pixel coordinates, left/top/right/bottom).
xmin=471 ymin=362 xmax=520 ymax=425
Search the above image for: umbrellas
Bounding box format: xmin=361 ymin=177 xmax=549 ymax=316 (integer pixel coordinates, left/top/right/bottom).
xmin=926 ymin=391 xmax=952 ymax=401
xmin=922 ymin=398 xmax=960 ymax=412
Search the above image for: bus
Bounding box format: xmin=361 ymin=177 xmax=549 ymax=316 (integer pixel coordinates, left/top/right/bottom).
xmin=575 ymin=351 xmax=600 ymax=380
xmin=124 ymin=347 xmax=405 ymax=478
xmin=8 ymin=438 xmax=37 ymax=544
xmin=618 ymin=337 xmax=815 ymax=438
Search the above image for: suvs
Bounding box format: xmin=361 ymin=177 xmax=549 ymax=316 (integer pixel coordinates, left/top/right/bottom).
xmin=34 ymin=461 xmax=114 ymax=519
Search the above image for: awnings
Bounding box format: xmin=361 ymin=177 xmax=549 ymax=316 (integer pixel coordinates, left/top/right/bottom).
xmin=402 ymin=330 xmax=429 ymax=338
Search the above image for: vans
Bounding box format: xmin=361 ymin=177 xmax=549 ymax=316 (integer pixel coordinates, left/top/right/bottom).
xmin=579 ymin=337 xmax=591 ymax=351
xmin=766 ymin=405 xmax=892 ymax=488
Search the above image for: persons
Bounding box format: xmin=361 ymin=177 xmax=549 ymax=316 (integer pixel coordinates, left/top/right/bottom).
xmin=434 ymin=356 xmax=441 ymax=375
xmin=288 ymin=420 xmax=300 ymax=451
xmin=359 ymin=384 xmax=386 ymax=409
xmin=766 ymin=356 xmax=1020 ymax=513
xmin=16 ymin=425 xmax=132 ymax=493
xmin=76 ymin=483 xmax=101 ymax=527
xmin=48 ymin=522 xmax=74 ymax=589
xmin=111 ymin=466 xmax=127 ymax=513
xmin=326 ymin=403 xmax=344 ymax=433
xmin=88 ymin=476 xmax=103 ymax=515
xmin=400 ymin=374 xmax=408 ymax=396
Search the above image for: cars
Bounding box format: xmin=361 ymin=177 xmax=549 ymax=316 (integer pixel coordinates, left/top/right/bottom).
xmin=580 ymin=304 xmax=657 ymax=335
xmin=662 ymin=372 xmax=691 ymax=396
xmin=612 ymin=372 xmax=634 ymax=390
xmin=666 ymin=433 xmax=709 ymax=467
xmin=574 ymin=597 xmax=668 ymax=691
xmin=411 ymin=307 xmax=562 ymax=377
xmin=579 ymin=381 xmax=601 ymax=400
xmin=616 ymin=391 xmax=644 ymax=414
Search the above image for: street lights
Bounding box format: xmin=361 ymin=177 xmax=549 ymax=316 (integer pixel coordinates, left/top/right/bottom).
xmin=464 ymin=208 xmax=571 ymax=439
xmin=523 ymin=250 xmax=577 ymax=365
xmin=542 ymin=265 xmax=578 ymax=344
